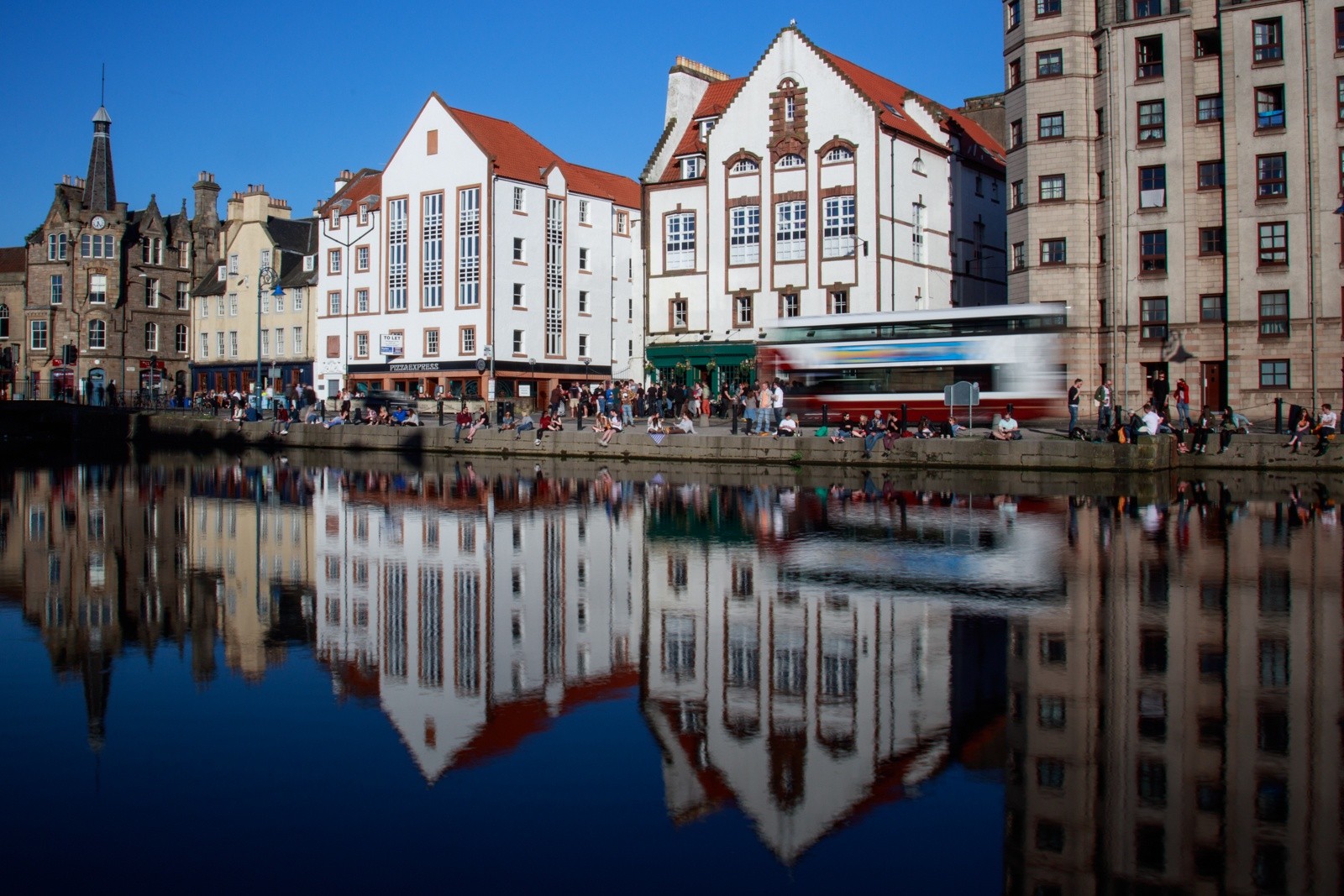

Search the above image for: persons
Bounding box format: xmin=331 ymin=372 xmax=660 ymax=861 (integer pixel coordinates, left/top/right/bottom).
xmin=78 ymin=359 xmax=1342 ymax=457
xmin=826 ymin=468 xmax=1334 ymax=529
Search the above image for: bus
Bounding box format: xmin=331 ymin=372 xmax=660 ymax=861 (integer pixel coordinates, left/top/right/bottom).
xmin=759 ymin=303 xmax=1071 ymax=427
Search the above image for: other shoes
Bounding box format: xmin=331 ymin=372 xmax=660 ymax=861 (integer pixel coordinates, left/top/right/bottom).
xmin=515 ymin=434 xmax=521 ymax=438
xmin=887 ymin=451 xmax=891 ymax=455
xmin=1201 ymin=444 xmax=1206 ymax=452
xmin=773 ymin=436 xmax=779 ymax=440
xmin=839 ymin=438 xmax=845 ymax=442
xmin=599 ymin=439 xmax=608 ymax=446
xmin=1182 ymin=446 xmax=1190 ymax=451
xmin=1177 ymin=447 xmax=1186 ymax=453
xmin=1223 ymin=446 xmax=1228 ymax=451
xmin=1194 ymin=444 xmax=1199 ymax=450
xmin=280 ymin=430 xmax=288 ymax=434
xmin=534 ymin=439 xmax=541 ymax=445
xmin=324 ymin=424 xmax=329 ymax=429
xmin=862 ymin=451 xmax=872 ymax=458
xmin=464 ymin=437 xmax=472 ymax=443
xmin=885 ymin=450 xmax=887 ymax=453
xmin=831 ymin=436 xmax=836 ymax=443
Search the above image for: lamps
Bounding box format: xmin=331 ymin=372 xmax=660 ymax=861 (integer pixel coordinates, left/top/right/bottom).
xmin=850 ymin=234 xmax=868 ymax=256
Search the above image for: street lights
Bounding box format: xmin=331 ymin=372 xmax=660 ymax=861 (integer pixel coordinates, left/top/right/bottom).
xmin=256 ymin=265 xmax=287 ymax=420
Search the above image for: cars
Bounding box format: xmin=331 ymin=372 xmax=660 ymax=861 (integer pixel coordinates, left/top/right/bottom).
xmin=365 ymin=390 xmax=418 ymax=415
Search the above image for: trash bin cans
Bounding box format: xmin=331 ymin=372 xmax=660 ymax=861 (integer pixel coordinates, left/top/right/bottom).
xmin=184 ymin=398 xmax=191 ymax=407
xmin=497 ymin=401 xmax=512 ymax=426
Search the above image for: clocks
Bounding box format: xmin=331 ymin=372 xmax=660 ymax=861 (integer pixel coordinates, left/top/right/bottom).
xmin=92 ymin=215 xmax=106 ymax=230
